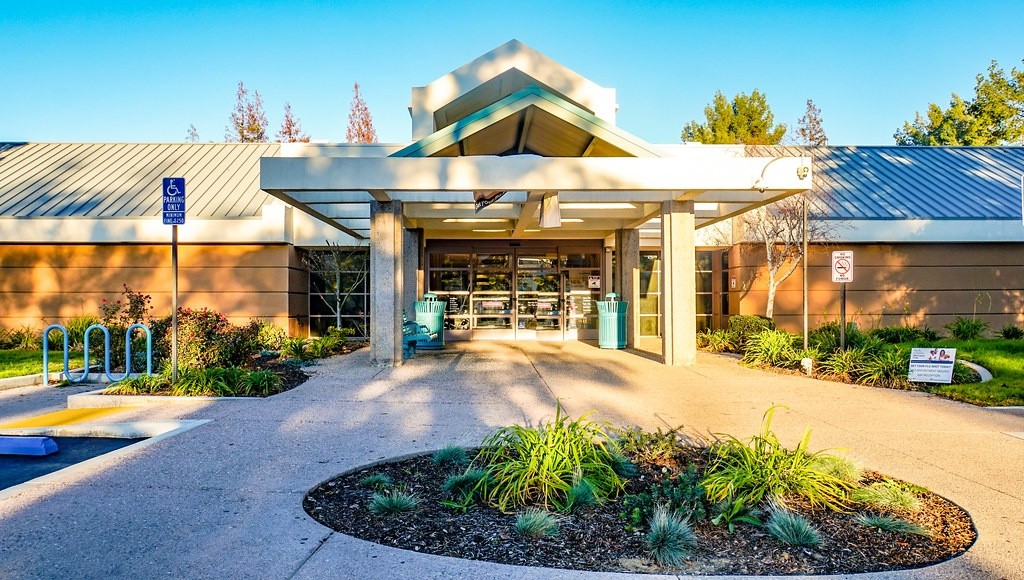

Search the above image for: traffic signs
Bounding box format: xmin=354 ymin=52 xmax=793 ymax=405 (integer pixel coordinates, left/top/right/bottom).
xmin=162 ymin=177 xmax=186 ymax=225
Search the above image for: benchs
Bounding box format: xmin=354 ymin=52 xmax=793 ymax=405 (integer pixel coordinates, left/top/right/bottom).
xmin=402 ymin=310 xmax=438 ymax=361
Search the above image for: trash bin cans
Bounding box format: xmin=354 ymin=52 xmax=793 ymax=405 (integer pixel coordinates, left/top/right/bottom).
xmin=414 ymin=293 xmax=447 ymax=350
xmin=596 ymin=293 xmax=630 ymax=350
